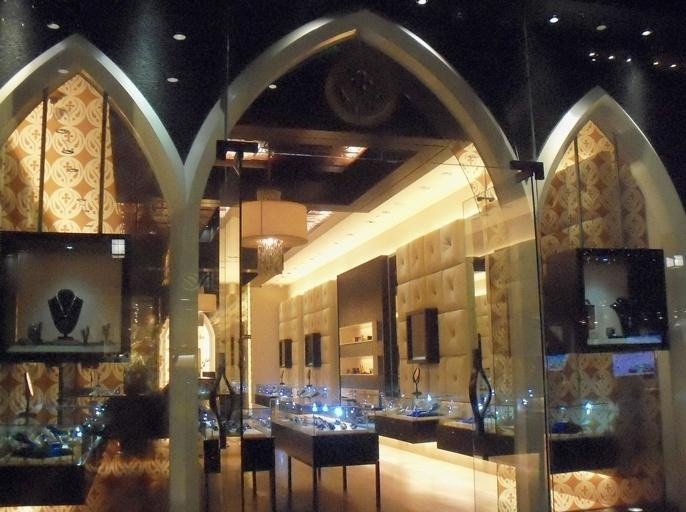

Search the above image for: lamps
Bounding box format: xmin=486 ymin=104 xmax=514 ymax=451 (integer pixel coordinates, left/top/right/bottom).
xmin=240 ymin=200 xmax=308 ymax=276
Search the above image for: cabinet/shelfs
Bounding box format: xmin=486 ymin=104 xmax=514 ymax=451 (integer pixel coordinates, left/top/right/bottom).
xmin=278 ymin=339 xmax=292 ymax=368
xmin=305 ymin=332 xmax=321 ymax=367
xmin=0 ymin=230 xmax=131 ymax=369
xmin=405 ymin=308 xmax=440 ymax=363
xmin=544 ymin=246 xmax=670 ymax=355
xmin=339 ymin=320 xmax=380 ymax=409
xmin=0 ymin=402 xmax=110 ymax=506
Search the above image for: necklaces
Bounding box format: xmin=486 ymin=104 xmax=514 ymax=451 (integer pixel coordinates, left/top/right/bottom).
xmin=55 ymin=294 xmax=78 ymax=322
xmin=618 ymin=303 xmax=635 ymax=329
